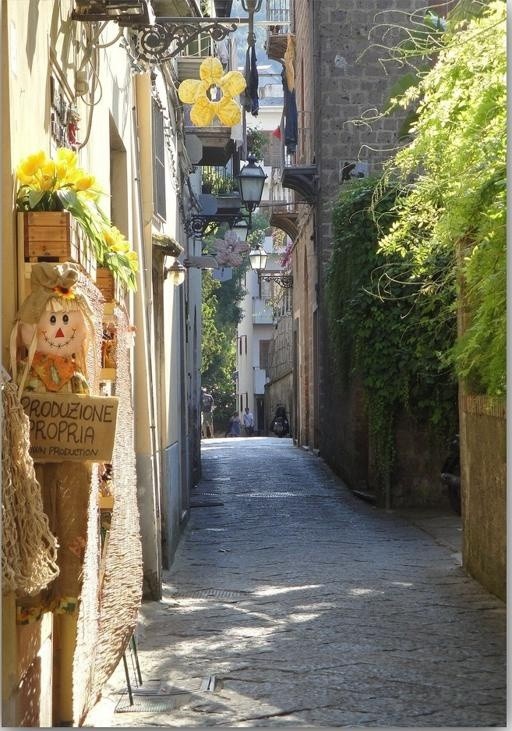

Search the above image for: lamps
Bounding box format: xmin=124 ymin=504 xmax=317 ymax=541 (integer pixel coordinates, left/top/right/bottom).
xmin=164 ymin=259 xmax=186 ymax=287
xmin=182 ymin=151 xmax=268 ymax=240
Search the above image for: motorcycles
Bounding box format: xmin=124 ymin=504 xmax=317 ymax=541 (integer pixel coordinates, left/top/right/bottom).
xmin=271 ymin=403 xmax=289 ymax=438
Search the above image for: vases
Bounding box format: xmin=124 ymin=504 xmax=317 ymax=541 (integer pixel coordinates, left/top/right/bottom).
xmin=96 ymin=268 xmax=126 ymax=304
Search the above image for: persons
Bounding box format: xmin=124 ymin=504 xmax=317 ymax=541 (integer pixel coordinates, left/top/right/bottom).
xmin=201 ymin=388 xmax=215 ymax=438
xmin=7 ymin=258 xmax=107 ymax=395
xmin=243 ymin=407 xmax=256 ymax=439
xmin=228 ymin=411 xmax=240 ymax=438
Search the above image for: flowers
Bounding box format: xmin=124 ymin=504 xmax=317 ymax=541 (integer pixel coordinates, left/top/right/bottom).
xmin=14 ymin=148 xmax=140 ymax=292
